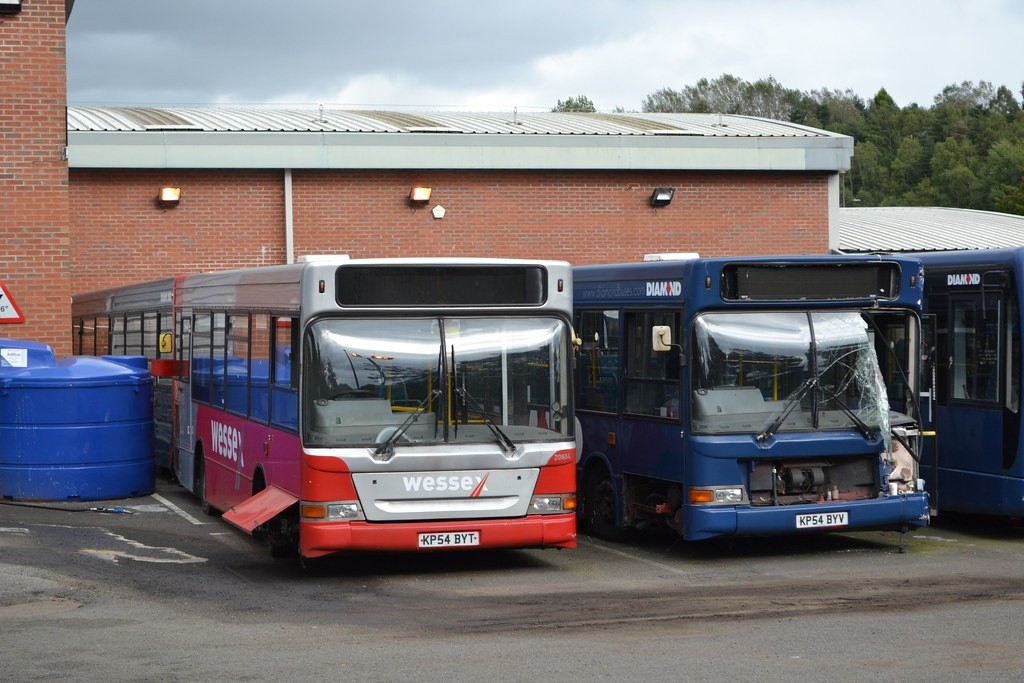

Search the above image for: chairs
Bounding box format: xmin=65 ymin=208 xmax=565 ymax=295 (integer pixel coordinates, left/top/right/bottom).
xmin=389 ymin=398 xmax=423 ymax=414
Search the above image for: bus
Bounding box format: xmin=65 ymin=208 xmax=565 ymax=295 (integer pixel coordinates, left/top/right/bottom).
xmin=70 ymin=255 xmax=589 ymax=554
xmin=556 ymin=250 xmax=934 ymax=535
xmin=865 ymin=248 xmax=1024 ymax=519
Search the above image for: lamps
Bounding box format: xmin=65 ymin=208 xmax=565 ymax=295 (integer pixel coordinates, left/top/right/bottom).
xmin=649 ymin=186 xmax=675 ymax=207
xmin=156 ymin=186 xmax=181 ymax=208
xmin=407 ymin=187 xmax=432 ymax=207
